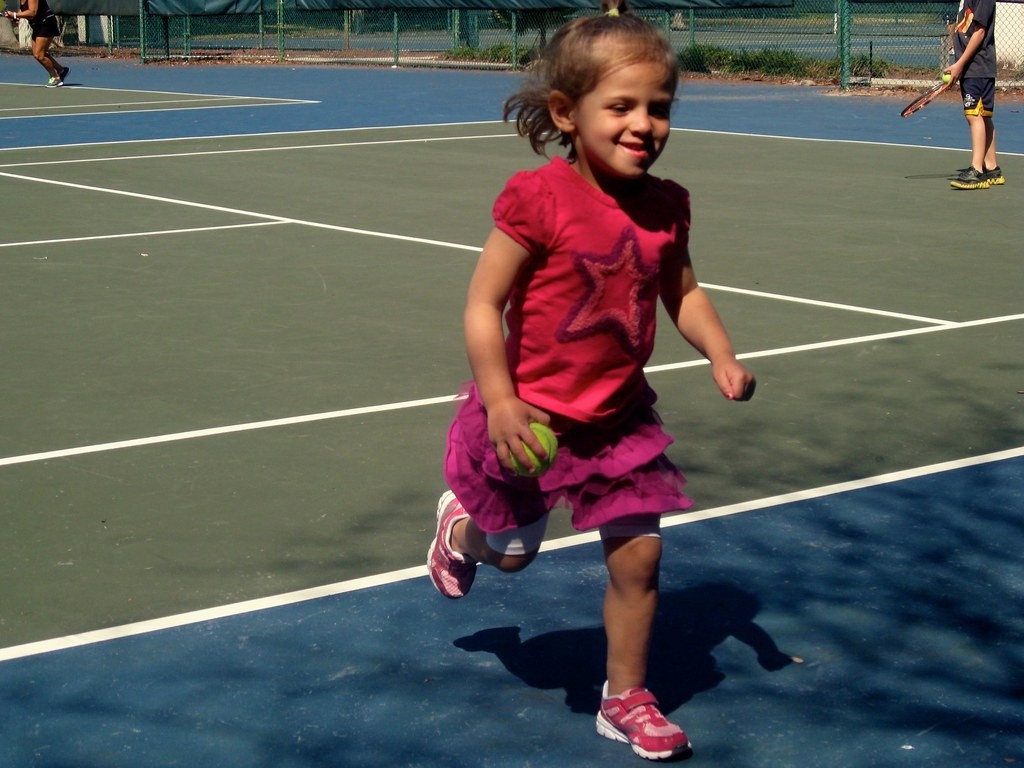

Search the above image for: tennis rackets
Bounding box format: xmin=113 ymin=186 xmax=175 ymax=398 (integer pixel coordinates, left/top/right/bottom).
xmin=900 ymin=83 xmax=954 ymax=116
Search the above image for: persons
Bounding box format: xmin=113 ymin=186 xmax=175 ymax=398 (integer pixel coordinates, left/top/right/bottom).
xmin=425 ymin=0 xmax=757 ymax=763
xmin=3 ymin=0 xmax=71 ymax=89
xmin=947 ymin=0 xmax=1005 ymax=189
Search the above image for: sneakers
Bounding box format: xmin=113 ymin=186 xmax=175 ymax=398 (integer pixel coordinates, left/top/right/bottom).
xmin=986 ymin=166 xmax=1005 ymax=185
xmin=44 ymin=74 xmax=64 ymax=88
xmin=596 ymin=680 xmax=693 ymax=761
xmin=950 ymin=166 xmax=990 ymax=190
xmin=427 ymin=490 xmax=483 ymax=599
xmin=58 ymin=67 xmax=70 ymax=81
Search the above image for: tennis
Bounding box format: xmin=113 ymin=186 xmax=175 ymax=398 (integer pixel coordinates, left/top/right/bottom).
xmin=942 ymin=71 xmax=952 ymax=83
xmin=511 ymin=424 xmax=559 ymax=476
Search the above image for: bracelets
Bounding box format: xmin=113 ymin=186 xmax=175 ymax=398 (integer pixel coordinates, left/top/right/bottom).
xmin=14 ymin=12 xmax=16 ymax=18
